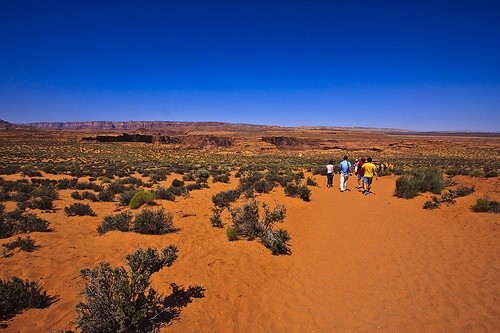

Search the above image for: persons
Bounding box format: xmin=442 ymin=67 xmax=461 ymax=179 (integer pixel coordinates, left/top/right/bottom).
xmin=362 ymin=157 xmax=378 ymax=196
xmin=357 ymin=157 xmax=366 ymax=189
xmin=325 ymin=161 xmax=334 ymax=188
xmin=338 ymin=155 xmax=352 ymax=192
xmin=354 ymin=159 xmax=360 ymax=175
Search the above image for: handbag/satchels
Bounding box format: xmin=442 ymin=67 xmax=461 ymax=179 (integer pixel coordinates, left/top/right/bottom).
xmin=347 ymin=165 xmax=349 ymax=174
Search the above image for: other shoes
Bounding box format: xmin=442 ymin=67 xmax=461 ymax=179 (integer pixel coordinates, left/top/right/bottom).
xmin=328 ymin=185 xmax=333 ymax=188
xmin=358 ymin=187 xmax=363 ymax=190
xmin=340 ymin=190 xmax=343 ymax=192
xmin=364 ymin=191 xmax=369 ymax=195
